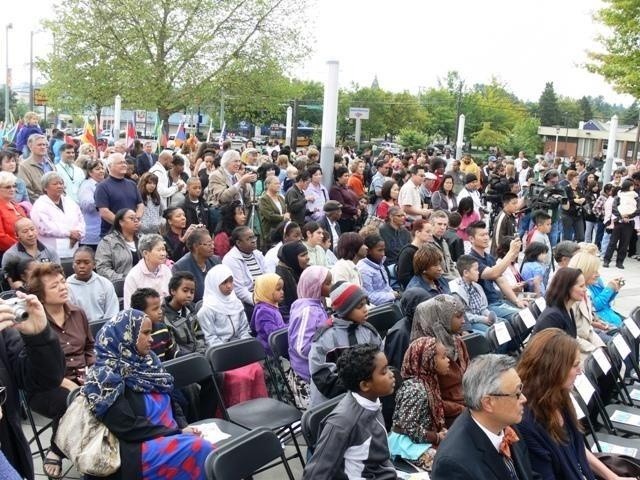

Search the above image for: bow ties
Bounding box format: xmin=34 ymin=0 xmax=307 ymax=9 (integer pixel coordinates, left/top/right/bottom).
xmin=500 ymin=424 xmax=520 ymax=458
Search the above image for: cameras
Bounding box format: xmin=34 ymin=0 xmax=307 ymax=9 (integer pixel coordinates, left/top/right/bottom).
xmin=244 ymin=166 xmax=267 ymax=183
xmin=618 ymin=279 xmax=624 ymax=287
xmin=2 ymin=297 xmax=28 ymax=324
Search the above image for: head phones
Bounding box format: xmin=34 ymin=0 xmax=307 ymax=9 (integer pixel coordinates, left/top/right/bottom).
xmin=543 ymin=170 xmax=552 ymax=183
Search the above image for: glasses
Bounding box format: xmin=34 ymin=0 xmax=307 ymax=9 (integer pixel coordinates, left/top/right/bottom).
xmin=248 ymin=236 xmax=258 ymax=242
xmin=489 ymin=383 xmax=524 ymax=398
xmin=199 ymin=240 xmax=214 ymax=247
xmin=2 ymin=185 xmax=17 ymax=188
xmin=131 ymin=216 xmax=140 ymax=221
xmin=394 ymin=215 xmax=405 ymax=217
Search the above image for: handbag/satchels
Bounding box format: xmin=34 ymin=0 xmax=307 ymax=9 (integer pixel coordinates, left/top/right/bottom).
xmin=54 ymin=396 xmax=121 ymax=477
xmin=592 ymin=452 xmax=640 ymax=480
xmin=363 ymin=202 xmax=386 ymax=230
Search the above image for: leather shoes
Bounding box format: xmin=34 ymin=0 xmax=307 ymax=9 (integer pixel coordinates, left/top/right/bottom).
xmin=616 ymin=265 xmax=624 ymax=269
xmin=603 ymin=261 xmax=609 ymax=267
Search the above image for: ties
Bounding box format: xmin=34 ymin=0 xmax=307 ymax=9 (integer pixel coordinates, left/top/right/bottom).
xmin=232 ymin=176 xmax=240 ymax=200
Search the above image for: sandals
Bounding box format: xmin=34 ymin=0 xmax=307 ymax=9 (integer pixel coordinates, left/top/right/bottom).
xmin=43 ymin=446 xmax=62 ymax=479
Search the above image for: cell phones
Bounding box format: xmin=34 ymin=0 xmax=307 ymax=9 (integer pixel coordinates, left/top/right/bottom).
xmin=514 ymin=233 xmax=520 ymax=240
xmin=41 ymin=258 xmax=50 ymax=263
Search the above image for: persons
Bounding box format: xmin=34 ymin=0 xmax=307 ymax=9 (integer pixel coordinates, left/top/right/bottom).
xmin=1 ymin=110 xmax=640 ymax=480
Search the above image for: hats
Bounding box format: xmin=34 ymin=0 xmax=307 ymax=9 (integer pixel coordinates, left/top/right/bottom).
xmin=488 ymin=156 xmax=498 ymax=162
xmin=424 ymin=172 xmax=438 ymax=180
xmin=323 ymin=200 xmax=343 ymax=211
xmin=466 ymin=173 xmax=477 ymax=184
xmin=328 ymin=281 xmax=368 ymax=318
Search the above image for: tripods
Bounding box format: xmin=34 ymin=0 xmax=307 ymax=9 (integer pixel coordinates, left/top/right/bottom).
xmin=245 ymin=183 xmax=264 ymax=235
